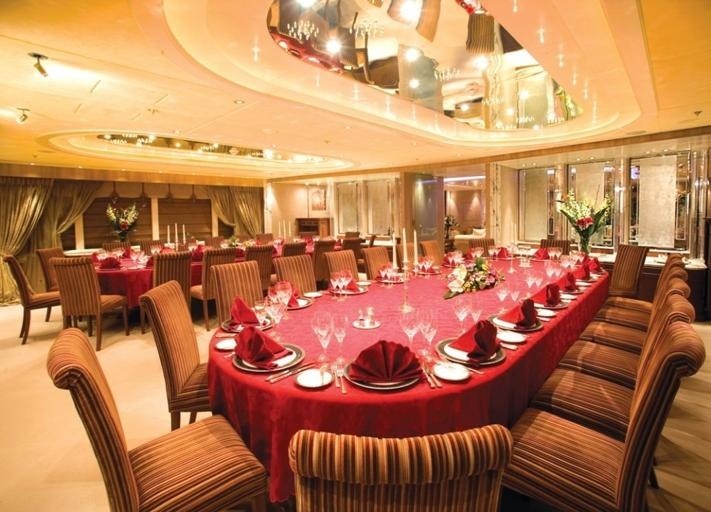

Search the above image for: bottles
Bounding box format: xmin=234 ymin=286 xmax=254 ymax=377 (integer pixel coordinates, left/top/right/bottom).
xmin=357 ymin=305 xmax=375 ymax=327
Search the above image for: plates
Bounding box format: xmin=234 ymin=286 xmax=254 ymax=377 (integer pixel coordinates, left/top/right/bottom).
xmin=492 ymin=272 xmax=600 ymax=332
xmin=288 ymin=262 xmax=457 ymax=312
xmin=435 ymin=336 xmax=505 ymax=365
xmin=231 ymin=342 xmax=303 ymax=373
xmin=494 ymin=330 xmax=526 ymax=343
xmin=351 ymin=318 xmax=381 ymax=330
xmin=220 ymin=316 xmax=275 ymax=333
xmin=213 ymin=337 xmax=237 ymax=351
xmin=344 ymin=360 xmax=418 ymax=390
xmin=299 ymin=368 xmax=333 ymax=389
xmin=433 ymin=360 xmax=470 ymax=381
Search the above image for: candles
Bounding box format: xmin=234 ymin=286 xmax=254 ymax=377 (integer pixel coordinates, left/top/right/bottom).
xmin=276 ymin=218 xmax=293 ymax=237
xmin=165 ymin=223 xmax=188 ymax=244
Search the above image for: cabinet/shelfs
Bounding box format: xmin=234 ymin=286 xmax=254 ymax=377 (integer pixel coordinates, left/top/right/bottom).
xmin=295 ymin=216 xmax=330 ymax=238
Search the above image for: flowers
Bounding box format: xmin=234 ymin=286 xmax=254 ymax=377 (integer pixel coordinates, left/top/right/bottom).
xmin=551 ymin=181 xmax=620 ymax=255
xmin=105 ymin=201 xmax=144 ymax=229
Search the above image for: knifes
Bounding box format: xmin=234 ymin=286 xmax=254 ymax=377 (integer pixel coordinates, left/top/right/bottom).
xmin=420 ymin=363 xmax=441 ymax=388
xmin=265 ymin=360 xmax=318 ymax=385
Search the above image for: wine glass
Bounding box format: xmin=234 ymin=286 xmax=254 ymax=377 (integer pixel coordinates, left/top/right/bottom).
xmin=329 ymin=253 xmax=438 ymax=302
xmin=254 ymin=281 xmax=292 ymax=337
xmin=308 ymin=309 xmax=350 ymax=364
xmin=445 ymin=240 xmax=585 ymax=336
xmin=91 ymin=243 xmax=162 ymax=270
xmin=398 ymin=306 xmax=438 ymax=362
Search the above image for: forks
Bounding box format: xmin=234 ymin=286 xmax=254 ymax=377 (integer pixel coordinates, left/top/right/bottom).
xmin=330 ymin=363 xmax=347 ymax=397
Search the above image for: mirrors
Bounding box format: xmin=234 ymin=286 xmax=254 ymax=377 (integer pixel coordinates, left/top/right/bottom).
xmin=262 ymin=0 xmax=585 ymax=134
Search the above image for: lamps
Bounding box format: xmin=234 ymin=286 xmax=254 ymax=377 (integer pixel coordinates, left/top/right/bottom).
xmin=35 ymin=55 xmax=51 ymax=78
xmin=12 ymin=106 xmax=33 ymax=127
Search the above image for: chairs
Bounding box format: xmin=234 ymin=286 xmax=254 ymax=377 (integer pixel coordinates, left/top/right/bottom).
xmin=272 ymin=3 xmax=520 ymax=91
xmin=1 ymin=254 xmax=61 ymax=347
xmin=36 ymin=246 xmax=66 ymax=324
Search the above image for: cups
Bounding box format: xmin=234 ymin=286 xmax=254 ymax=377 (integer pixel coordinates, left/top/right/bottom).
xmin=291 ymin=234 xmax=323 ymax=243
